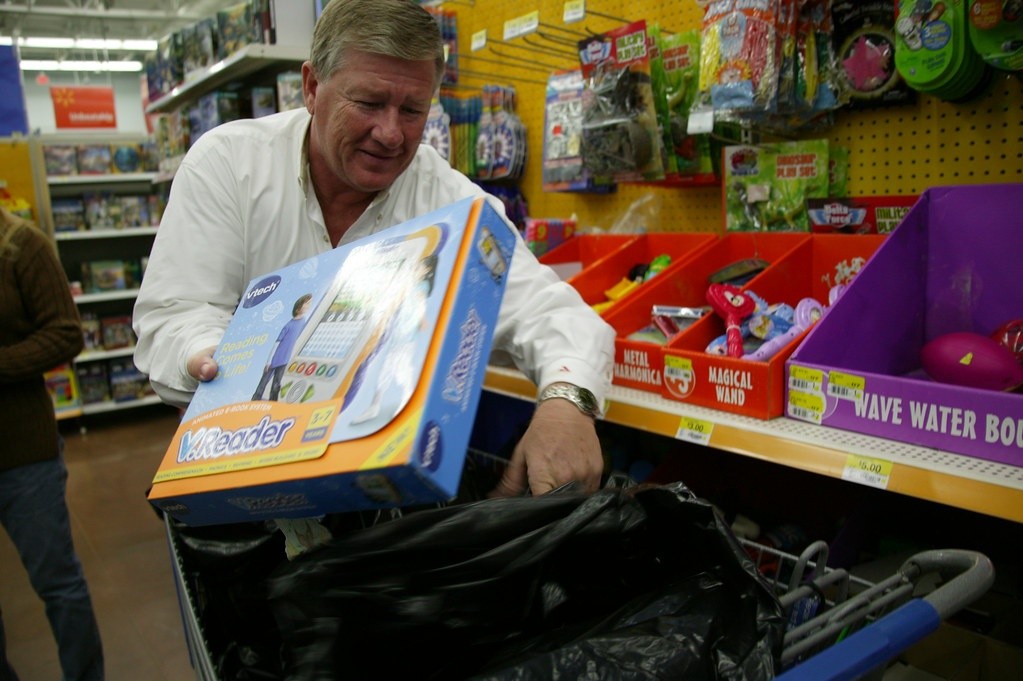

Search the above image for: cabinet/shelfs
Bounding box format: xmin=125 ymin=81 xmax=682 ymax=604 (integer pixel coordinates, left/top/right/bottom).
xmin=26 ymin=130 xmax=179 ymax=431
xmin=309 ymin=0 xmax=1023 ymax=628
xmin=142 ymin=1 xmax=314 ymax=182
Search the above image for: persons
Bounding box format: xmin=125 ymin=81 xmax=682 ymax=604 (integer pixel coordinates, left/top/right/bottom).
xmin=351 ymin=255 xmax=439 ymax=423
xmin=1 ymin=210 xmax=104 ymax=681
xmin=133 ymin=0 xmax=616 ymax=496
xmin=251 ymin=293 xmax=313 ymax=402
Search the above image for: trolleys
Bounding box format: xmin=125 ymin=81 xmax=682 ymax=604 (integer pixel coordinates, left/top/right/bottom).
xmin=159 ymin=439 xmax=996 ymax=681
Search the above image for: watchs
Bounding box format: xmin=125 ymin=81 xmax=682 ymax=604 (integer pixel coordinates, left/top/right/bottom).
xmin=537 ymin=386 xmax=601 ymax=417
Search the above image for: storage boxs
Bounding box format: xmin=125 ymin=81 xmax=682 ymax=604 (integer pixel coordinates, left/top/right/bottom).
xmin=159 ymin=72 xmax=300 ymax=159
xmin=147 ymin=199 xmax=518 ymax=530
xmin=533 ymin=177 xmax=1023 ymax=471
xmin=142 ymin=1 xmax=270 ymax=102
xmin=40 ymin=145 xmax=166 ymax=410
